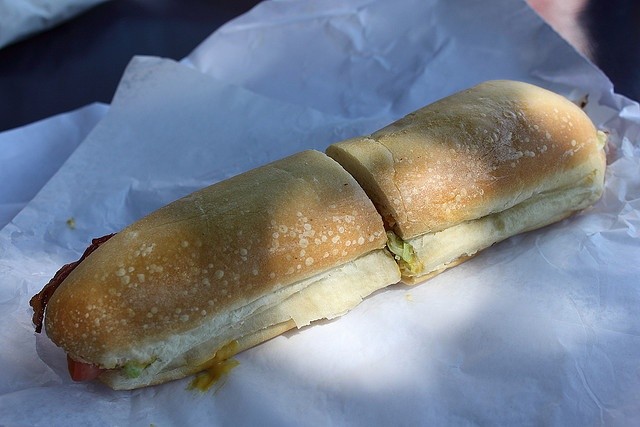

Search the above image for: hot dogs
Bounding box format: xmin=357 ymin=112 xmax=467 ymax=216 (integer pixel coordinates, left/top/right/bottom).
xmin=30 ymin=149 xmax=401 ymax=391
xmin=324 ymin=80 xmax=607 ymax=285
